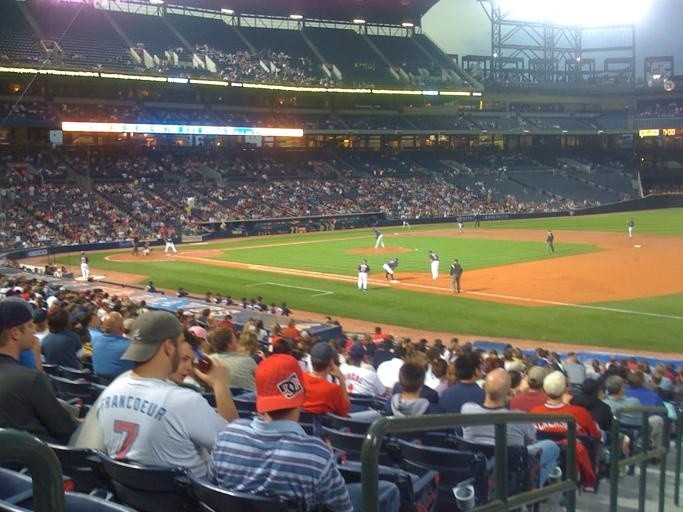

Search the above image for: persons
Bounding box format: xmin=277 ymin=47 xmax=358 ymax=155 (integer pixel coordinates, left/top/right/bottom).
xmin=450 ymin=259 xmax=462 ymax=294
xmin=289 ymin=341 xmax=352 ymax=418
xmin=357 ymin=326 xmax=683 ymax=511
xmin=1 ymin=250 xmax=378 ymax=382
xmin=68 ymin=332 xmax=198 ymax=487
xmin=96 ymin=311 xmax=240 ymax=491
xmin=209 ymin=354 xmax=356 ymax=512
xmin=428 ymin=250 xmax=440 ymax=280
xmin=383 ymin=258 xmax=400 ymax=280
xmin=0 ymin=31 xmax=682 ymax=252
xmin=1 ymin=296 xmax=82 ymax=448
xmin=340 ymin=341 xmax=387 ymax=396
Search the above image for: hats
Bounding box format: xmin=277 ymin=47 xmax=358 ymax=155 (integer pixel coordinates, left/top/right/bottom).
xmin=253 ymin=352 xmax=307 ymax=415
xmin=120 ymin=308 xmax=182 ymax=364
xmin=187 ymin=326 xmax=210 ymax=346
xmin=1 ymin=297 xmax=35 ymax=331
xmin=541 ymin=370 xmax=566 ymax=397
xmin=527 ymin=364 xmax=546 ymax=385
xmin=345 ymin=343 xmax=363 ymax=359
xmin=309 ymin=341 xmax=338 ymax=361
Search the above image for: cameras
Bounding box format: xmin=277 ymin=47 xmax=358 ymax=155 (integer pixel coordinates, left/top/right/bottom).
xmin=192 ymin=354 xmax=213 ymax=372
